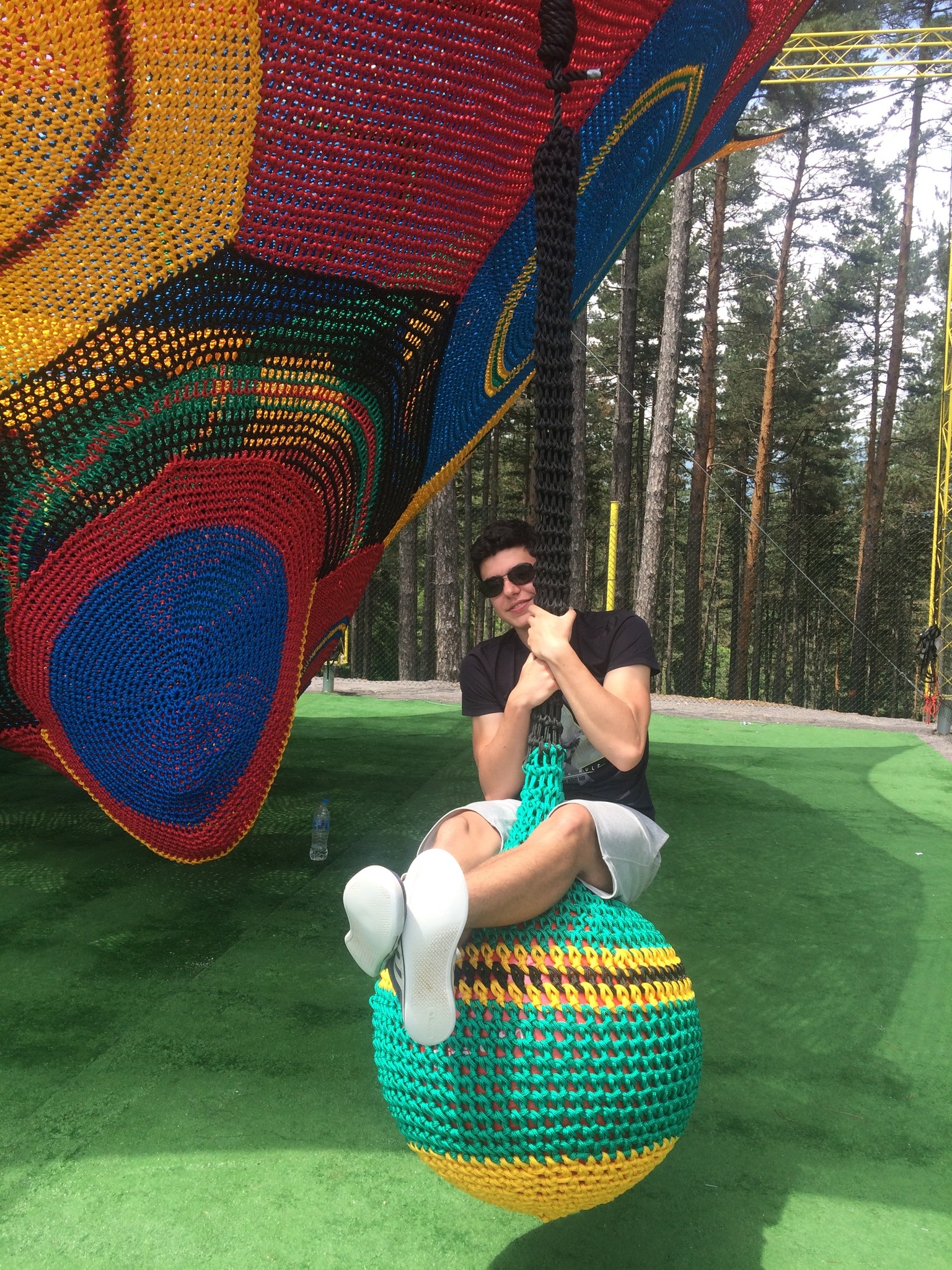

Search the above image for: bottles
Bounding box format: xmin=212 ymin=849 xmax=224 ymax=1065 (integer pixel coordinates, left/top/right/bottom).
xmin=309 ymin=799 xmax=330 ymax=861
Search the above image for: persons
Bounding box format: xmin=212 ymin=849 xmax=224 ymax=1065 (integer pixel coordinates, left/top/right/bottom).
xmin=340 ymin=517 xmax=670 ymax=1045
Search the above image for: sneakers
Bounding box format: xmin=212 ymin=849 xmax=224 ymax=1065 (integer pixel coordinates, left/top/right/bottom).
xmin=343 ymin=865 xmax=407 ymax=978
xmin=387 ymin=849 xmax=469 ymax=1047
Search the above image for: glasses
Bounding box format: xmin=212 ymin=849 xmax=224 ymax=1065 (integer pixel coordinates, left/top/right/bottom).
xmin=478 ymin=563 xmax=535 ymax=598
